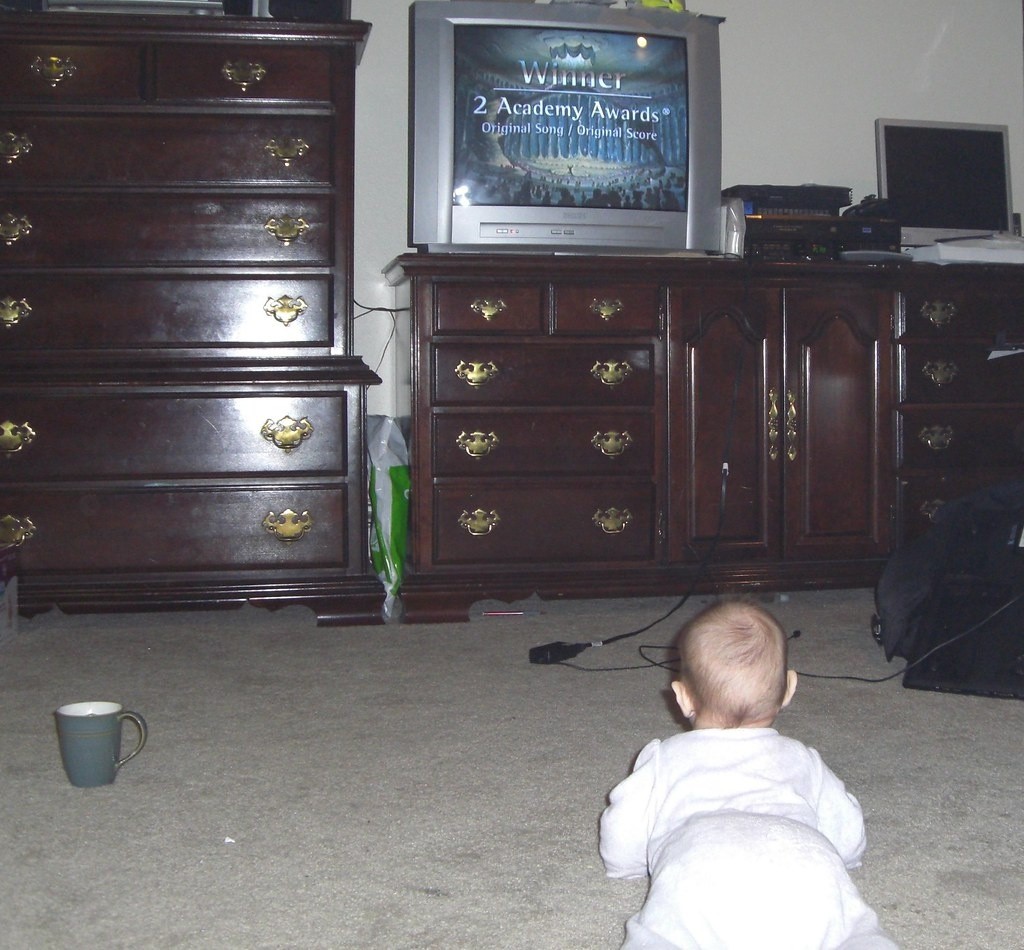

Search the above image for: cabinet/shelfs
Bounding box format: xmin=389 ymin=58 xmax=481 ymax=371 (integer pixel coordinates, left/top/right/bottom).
xmin=0 ymin=11 xmax=387 ymax=627
xmin=400 ymin=252 xmax=1023 ymax=622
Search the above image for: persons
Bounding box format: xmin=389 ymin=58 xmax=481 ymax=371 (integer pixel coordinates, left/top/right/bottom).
xmin=599 ymin=602 xmax=903 ymax=949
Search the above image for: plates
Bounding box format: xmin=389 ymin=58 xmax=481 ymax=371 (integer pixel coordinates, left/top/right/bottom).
xmin=841 ymin=251 xmax=913 ymax=263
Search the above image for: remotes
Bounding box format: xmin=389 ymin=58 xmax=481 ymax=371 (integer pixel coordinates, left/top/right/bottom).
xmin=840 ymin=250 xmax=913 ymax=262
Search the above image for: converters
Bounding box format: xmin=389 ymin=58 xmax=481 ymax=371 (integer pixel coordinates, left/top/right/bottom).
xmin=530 ymin=641 xmax=577 ymax=664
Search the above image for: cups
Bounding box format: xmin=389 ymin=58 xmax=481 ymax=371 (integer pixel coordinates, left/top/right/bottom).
xmin=54 ymin=701 xmax=147 ymax=787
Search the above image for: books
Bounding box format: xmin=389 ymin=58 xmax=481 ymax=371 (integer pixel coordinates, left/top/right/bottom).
xmin=906 ymin=242 xmax=1024 ymax=265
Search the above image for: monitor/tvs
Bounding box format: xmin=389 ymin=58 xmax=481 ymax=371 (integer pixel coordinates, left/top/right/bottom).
xmin=874 ymin=117 xmax=1014 ymax=245
xmin=407 ymin=0 xmax=720 ymax=256
xmin=904 ymin=574 xmax=1023 ymax=702
